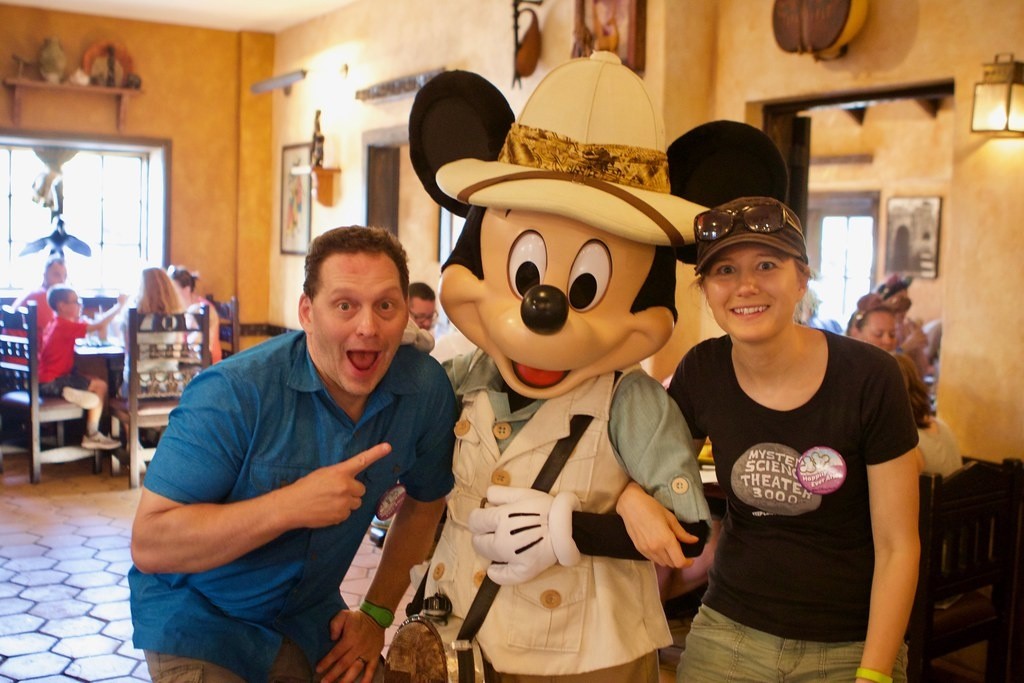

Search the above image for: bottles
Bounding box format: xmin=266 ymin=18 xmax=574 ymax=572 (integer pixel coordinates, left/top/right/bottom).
xmin=385 ymin=594 xmax=486 ymax=683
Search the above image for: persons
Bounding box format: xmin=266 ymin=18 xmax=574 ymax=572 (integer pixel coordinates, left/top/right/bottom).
xmin=2 ymin=257 xmax=129 ymax=451
xmin=615 ymin=196 xmax=919 ymax=683
xmin=794 ymin=282 xmax=843 ymax=334
xmin=127 ymin=225 xmax=461 ymax=683
xmin=115 ymin=265 xmax=222 ymax=473
xmin=399 ymin=282 xmax=438 ymax=353
xmin=846 ymin=288 xmax=963 ymax=481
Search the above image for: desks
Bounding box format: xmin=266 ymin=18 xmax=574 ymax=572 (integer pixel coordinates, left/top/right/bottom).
xmin=75 ymin=346 xmax=150 ymax=459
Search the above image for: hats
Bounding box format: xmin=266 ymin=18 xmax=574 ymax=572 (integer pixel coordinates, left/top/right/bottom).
xmin=694 ymin=196 xmax=808 ymax=277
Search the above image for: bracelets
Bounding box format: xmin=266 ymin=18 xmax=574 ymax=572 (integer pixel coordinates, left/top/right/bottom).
xmin=359 ymin=600 xmax=394 ymax=628
xmin=856 ymin=668 xmax=893 ymax=683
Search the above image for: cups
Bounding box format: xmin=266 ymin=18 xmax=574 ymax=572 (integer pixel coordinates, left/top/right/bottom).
xmin=94 ymin=313 xmax=109 ymax=344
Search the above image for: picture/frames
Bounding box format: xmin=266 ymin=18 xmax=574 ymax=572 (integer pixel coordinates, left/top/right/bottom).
xmin=281 ymin=143 xmax=311 ymax=256
xmin=571 ymin=0 xmax=647 ymax=78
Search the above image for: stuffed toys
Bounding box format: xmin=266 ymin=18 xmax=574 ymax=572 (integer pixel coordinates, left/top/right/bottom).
xmin=384 ymin=49 xmax=791 ymax=683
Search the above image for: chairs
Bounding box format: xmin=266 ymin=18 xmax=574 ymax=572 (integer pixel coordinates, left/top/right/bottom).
xmin=0 ymin=297 xmax=120 ymax=486
xmin=206 ymin=294 xmax=240 ymax=358
xmin=109 ymin=304 xmax=210 ymax=487
xmin=903 ymin=456 xmax=1023 ymax=682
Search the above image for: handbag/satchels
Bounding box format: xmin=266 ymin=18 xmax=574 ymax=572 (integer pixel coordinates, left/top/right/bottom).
xmin=384 ymin=599 xmax=488 ymax=683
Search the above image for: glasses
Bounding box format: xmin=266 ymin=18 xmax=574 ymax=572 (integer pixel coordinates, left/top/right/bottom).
xmin=408 ymin=308 xmax=439 ymax=322
xmin=695 ymin=205 xmax=802 ymax=242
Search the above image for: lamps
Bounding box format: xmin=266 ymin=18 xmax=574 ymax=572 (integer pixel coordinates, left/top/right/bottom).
xmin=250 ymin=69 xmax=305 ymax=94
xmin=886 ymin=196 xmax=942 ymax=279
xmin=970 ymin=51 xmax=1024 ymax=134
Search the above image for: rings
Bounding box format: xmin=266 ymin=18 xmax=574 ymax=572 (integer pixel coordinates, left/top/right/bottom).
xmin=356 ymin=657 xmax=368 ymax=666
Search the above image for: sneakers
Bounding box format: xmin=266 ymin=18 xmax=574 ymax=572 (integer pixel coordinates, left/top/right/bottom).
xmin=82 ymin=431 xmax=121 ymax=450
